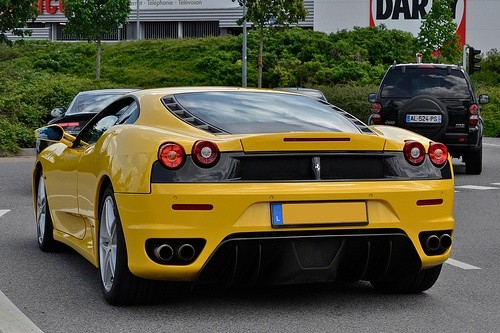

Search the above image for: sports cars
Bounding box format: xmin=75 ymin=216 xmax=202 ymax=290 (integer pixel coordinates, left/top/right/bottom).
xmin=31 ymin=86 xmax=455 ymax=304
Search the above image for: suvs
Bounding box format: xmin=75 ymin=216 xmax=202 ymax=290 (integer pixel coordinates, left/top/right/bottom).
xmin=366 ymin=63 xmax=492 ymax=176
